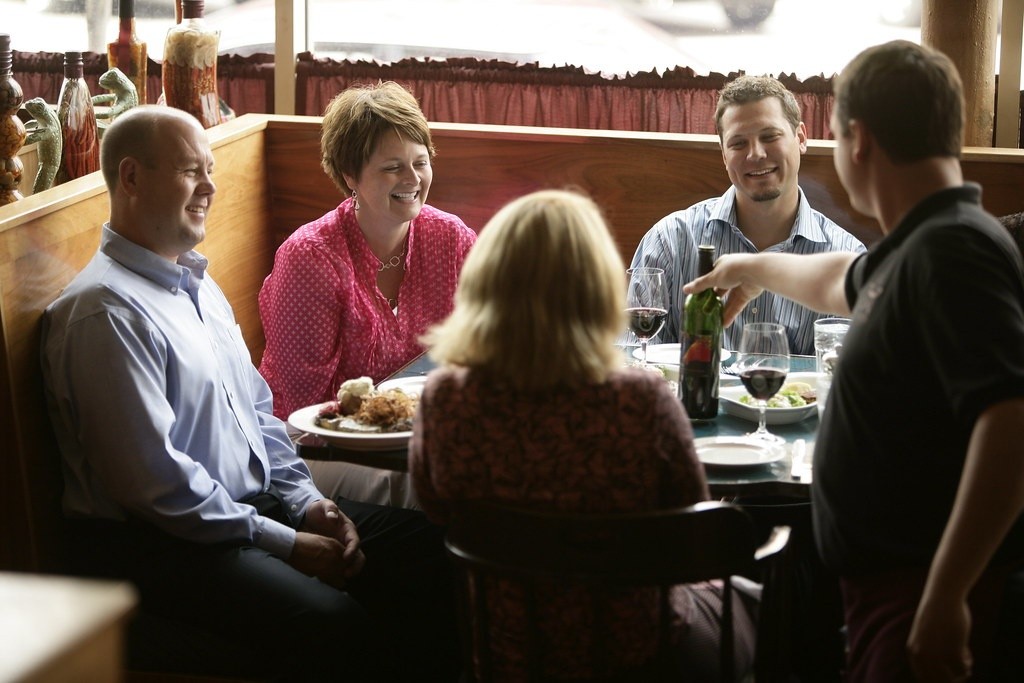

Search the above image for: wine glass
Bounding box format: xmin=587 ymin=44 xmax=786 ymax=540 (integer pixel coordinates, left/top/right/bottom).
xmin=626 ymin=267 xmax=669 ymax=366
xmin=737 ymin=322 xmax=790 ymax=435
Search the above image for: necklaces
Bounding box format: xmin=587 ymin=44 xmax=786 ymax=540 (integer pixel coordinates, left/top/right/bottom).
xmin=368 ymin=238 xmax=408 ymax=271
xmin=386 ymin=297 xmax=398 ymax=309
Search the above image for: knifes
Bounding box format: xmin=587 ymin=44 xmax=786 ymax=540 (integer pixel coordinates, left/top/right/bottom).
xmin=791 ymin=438 xmax=806 ymax=478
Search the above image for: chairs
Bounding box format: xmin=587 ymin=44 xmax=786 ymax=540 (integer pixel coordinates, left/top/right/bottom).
xmin=445 ymin=498 xmax=752 ymax=683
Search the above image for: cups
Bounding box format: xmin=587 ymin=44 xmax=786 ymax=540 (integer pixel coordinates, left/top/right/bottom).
xmin=813 ymin=317 xmax=852 ymax=424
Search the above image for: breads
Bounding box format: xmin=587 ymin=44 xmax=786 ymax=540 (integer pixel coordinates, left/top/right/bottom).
xmin=317 ymin=417 xmax=382 ymax=433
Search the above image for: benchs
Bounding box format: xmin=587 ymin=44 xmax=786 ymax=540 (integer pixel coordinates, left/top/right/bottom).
xmin=1 ymin=113 xmax=1024 ymax=575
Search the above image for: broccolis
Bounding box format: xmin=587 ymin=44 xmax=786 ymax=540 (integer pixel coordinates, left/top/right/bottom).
xmin=337 ymin=376 xmax=373 ymax=404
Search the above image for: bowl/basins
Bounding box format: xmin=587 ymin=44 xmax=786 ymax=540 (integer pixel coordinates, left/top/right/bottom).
xmin=718 ymin=372 xmax=819 ymax=425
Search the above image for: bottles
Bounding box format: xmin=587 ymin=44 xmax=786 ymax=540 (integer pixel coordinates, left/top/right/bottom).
xmin=677 ymin=245 xmax=724 ymax=420
xmin=106 ymin=0 xmax=147 ymax=107
xmin=162 ymin=0 xmax=222 ymax=130
xmin=56 ymin=51 xmax=100 ymax=185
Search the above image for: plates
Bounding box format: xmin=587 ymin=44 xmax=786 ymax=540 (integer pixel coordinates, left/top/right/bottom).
xmin=693 ymin=435 xmax=787 ymax=466
xmin=377 ymin=376 xmax=427 ymax=400
xmin=631 ymin=342 xmax=732 ymax=365
xmin=288 ymin=400 xmax=414 ymax=451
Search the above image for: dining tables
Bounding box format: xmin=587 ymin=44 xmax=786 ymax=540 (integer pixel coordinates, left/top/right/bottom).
xmin=295 ymin=345 xmax=824 ymax=683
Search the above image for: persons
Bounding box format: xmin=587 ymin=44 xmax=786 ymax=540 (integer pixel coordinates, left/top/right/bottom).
xmin=41 ymin=39 xmax=1024 ymax=683
